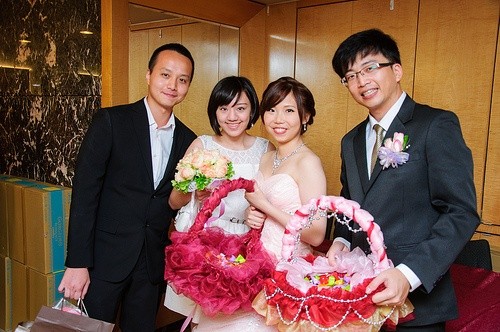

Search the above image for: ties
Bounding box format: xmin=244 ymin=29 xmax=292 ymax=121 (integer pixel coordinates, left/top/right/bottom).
xmin=371 ymin=124 xmax=385 ymax=174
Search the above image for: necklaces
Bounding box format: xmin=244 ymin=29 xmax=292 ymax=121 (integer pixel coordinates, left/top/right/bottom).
xmin=270 ymin=142 xmax=305 ymax=175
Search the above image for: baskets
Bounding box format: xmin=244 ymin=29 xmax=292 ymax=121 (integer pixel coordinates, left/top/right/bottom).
xmin=165 ymin=178 xmax=277 ymax=315
xmin=252 ymin=198 xmax=414 ymax=332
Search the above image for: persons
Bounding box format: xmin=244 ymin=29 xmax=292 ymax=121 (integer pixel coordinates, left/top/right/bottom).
xmin=163 ymin=76 xmax=276 ymax=332
xmin=191 ymin=76 xmax=327 ymax=332
xmin=332 ymin=28 xmax=482 ymax=332
xmin=58 ymin=43 xmax=197 ymax=332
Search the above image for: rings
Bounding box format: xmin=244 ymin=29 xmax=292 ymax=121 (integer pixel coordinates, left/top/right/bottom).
xmin=253 ymin=222 xmax=256 ymax=226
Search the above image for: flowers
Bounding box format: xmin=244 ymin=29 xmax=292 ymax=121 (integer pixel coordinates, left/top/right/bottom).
xmin=376 ymin=129 xmax=412 ymax=169
xmin=172 ymin=146 xmax=236 ymax=197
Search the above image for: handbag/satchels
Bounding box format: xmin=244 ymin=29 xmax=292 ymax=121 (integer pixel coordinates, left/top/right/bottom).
xmin=15 ymin=293 xmax=115 ymax=332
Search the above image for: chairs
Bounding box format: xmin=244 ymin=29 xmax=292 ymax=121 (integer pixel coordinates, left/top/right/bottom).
xmin=454 ymin=239 xmax=493 ymax=271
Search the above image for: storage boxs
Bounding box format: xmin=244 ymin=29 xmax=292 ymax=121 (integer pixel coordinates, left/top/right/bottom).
xmin=0 ymin=173 xmax=72 ymax=332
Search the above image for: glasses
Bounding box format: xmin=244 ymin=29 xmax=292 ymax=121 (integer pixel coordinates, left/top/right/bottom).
xmin=340 ymin=61 xmax=396 ymax=87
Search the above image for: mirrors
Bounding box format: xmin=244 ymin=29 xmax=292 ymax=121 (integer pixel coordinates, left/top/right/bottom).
xmin=127 ymin=3 xmax=240 ymax=138
